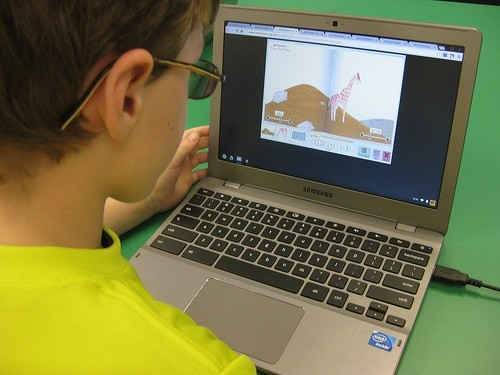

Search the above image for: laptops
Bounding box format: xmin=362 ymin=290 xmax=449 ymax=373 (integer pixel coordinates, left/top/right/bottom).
xmin=129 ymin=4 xmax=483 ymax=375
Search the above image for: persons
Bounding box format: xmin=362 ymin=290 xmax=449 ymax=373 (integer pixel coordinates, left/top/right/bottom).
xmin=0 ymin=0 xmax=257 ymax=375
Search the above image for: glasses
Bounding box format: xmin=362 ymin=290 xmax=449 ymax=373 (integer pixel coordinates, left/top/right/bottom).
xmin=60 ymin=57 xmax=226 ymax=131
